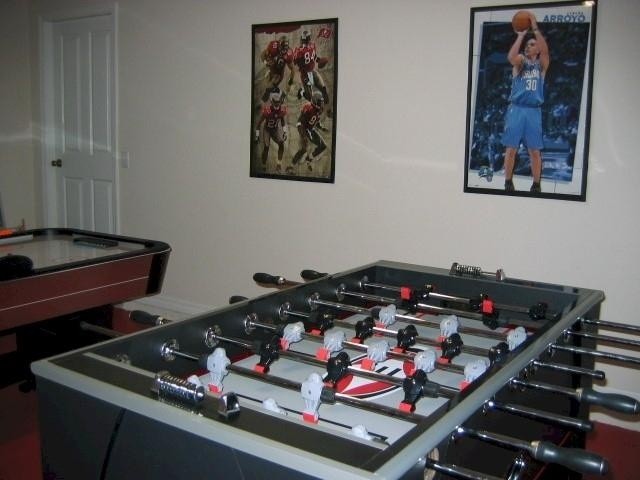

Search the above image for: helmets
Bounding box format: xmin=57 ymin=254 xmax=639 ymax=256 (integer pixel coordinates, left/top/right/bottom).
xmin=300 ymin=30 xmax=310 ymax=48
xmin=270 ymin=88 xmax=282 ymax=110
xmin=279 ymin=37 xmax=289 ymax=55
xmin=310 ymin=93 xmax=324 ymax=111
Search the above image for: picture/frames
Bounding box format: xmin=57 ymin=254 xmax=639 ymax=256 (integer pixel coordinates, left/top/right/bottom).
xmin=249 ymin=18 xmax=339 ymax=184
xmin=464 ymin=0 xmax=598 ymax=201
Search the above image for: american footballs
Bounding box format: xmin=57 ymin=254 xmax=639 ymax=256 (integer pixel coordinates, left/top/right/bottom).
xmin=318 ymin=58 xmax=329 ymax=68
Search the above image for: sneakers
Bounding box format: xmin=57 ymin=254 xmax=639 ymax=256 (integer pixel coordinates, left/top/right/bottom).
xmin=298 ymin=88 xmax=302 ymax=99
xmin=305 ymin=156 xmax=312 ymax=171
xmin=286 ymin=166 xmax=295 ymax=174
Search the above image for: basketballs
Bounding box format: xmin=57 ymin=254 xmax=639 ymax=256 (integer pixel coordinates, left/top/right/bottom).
xmin=512 ymin=10 xmax=532 ymax=32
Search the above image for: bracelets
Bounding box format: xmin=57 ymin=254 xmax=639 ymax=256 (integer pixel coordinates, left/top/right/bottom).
xmin=282 ymin=125 xmax=286 ymax=133
xmin=255 ymin=130 xmax=260 ymax=137
xmin=532 ymin=27 xmax=540 ymax=32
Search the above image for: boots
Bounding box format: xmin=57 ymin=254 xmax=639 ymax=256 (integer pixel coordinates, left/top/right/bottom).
xmin=275 ymin=164 xmax=281 ymax=175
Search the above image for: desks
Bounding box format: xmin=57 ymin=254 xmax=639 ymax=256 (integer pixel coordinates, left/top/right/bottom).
xmin=2 ymin=227 xmax=172 ymax=393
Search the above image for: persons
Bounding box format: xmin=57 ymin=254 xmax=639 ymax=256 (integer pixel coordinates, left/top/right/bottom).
xmin=285 ymin=91 xmax=330 ymax=176
xmin=260 ymin=36 xmax=295 ymax=103
xmin=291 ymin=31 xmax=331 ymax=106
xmin=252 ymin=87 xmax=288 ymax=174
xmin=499 ymin=12 xmax=550 ymax=194
